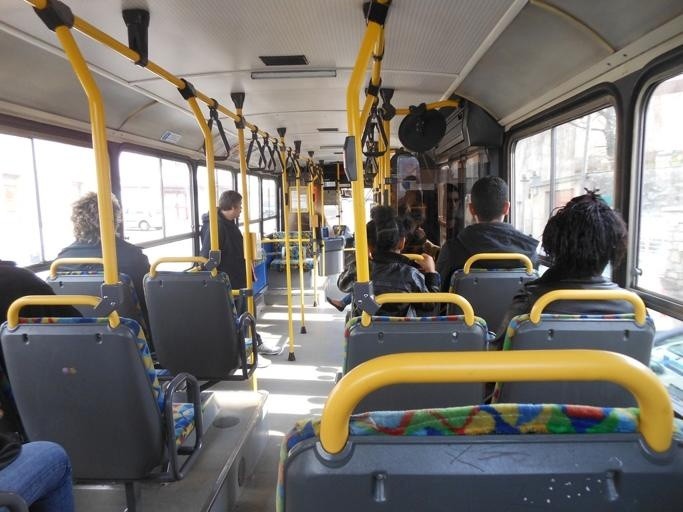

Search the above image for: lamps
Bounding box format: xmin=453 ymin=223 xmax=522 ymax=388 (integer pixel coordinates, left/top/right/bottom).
xmin=268 ymin=232 xmax=317 ymax=292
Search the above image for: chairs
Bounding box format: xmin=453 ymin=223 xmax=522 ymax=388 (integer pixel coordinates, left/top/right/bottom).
xmin=334 ymin=250 xmax=657 ymax=408
xmin=246 ymin=344 xmax=280 ymax=367
xmin=142 ymin=258 xmax=260 ymax=401
xmin=268 ymin=232 xmax=317 ymax=292
xmin=46 ymin=259 xmax=161 ymax=383
xmin=2 ymin=295 xmax=203 ymax=510
xmin=276 ymin=350 xmax=681 ymax=511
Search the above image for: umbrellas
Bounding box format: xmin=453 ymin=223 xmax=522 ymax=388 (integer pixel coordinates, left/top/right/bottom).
xmin=327 ymin=297 xmax=344 ymax=311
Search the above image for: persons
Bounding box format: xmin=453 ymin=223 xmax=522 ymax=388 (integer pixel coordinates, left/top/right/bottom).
xmin=0 ymin=441 xmax=75 ymax=512
xmin=200 ymin=191 xmax=281 ymax=355
xmin=55 ymin=193 xmax=151 ymax=335
xmin=485 ymin=187 xmax=647 ymax=404
xmin=328 ymin=183 xmax=470 ymax=312
xmin=435 ymin=175 xmax=539 ymax=317
xmin=0 ymin=260 xmax=84 ymax=317
xmin=365 ymin=205 xmax=440 ymax=317
